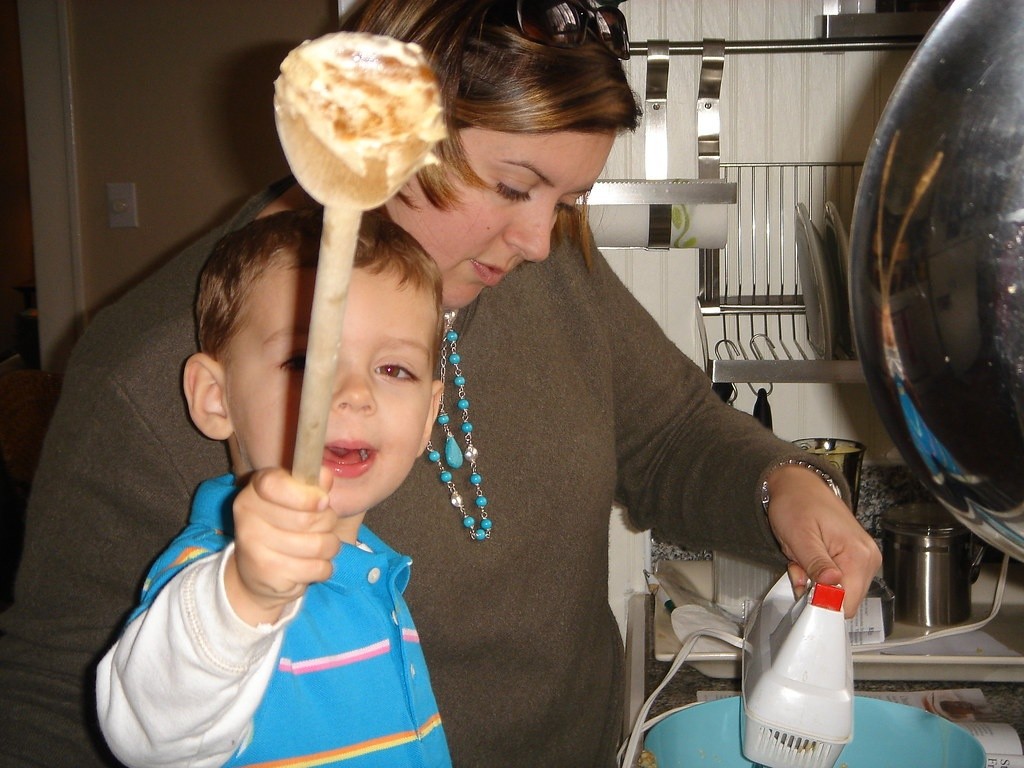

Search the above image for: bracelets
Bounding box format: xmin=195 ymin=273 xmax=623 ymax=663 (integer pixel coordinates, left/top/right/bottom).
xmin=761 ymin=460 xmax=843 ymax=514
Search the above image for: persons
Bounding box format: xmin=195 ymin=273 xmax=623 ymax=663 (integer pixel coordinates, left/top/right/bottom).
xmin=0 ymin=0 xmax=880 ymax=768
xmin=93 ymin=206 xmax=453 ymax=768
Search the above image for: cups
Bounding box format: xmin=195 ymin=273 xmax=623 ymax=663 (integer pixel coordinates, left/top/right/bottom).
xmin=882 ymin=500 xmax=986 ymax=628
xmin=791 ymin=438 xmax=866 ymax=522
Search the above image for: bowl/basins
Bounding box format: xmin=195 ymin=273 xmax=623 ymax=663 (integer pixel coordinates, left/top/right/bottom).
xmin=642 ymin=695 xmax=988 ymax=768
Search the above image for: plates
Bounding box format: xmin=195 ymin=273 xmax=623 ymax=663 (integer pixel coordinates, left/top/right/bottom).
xmin=820 ymin=198 xmax=855 ymax=361
xmin=793 ymin=201 xmax=843 ymax=361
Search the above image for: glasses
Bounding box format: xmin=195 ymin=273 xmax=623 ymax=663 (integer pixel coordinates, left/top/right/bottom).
xmin=518 ymin=0 xmax=630 ymax=60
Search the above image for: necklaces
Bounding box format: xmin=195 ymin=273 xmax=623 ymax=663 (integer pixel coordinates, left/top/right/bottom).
xmin=425 ymin=310 xmax=490 ymax=541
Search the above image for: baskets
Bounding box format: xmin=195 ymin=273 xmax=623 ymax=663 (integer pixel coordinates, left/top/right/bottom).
xmin=0 ymin=370 xmax=65 ymax=479
